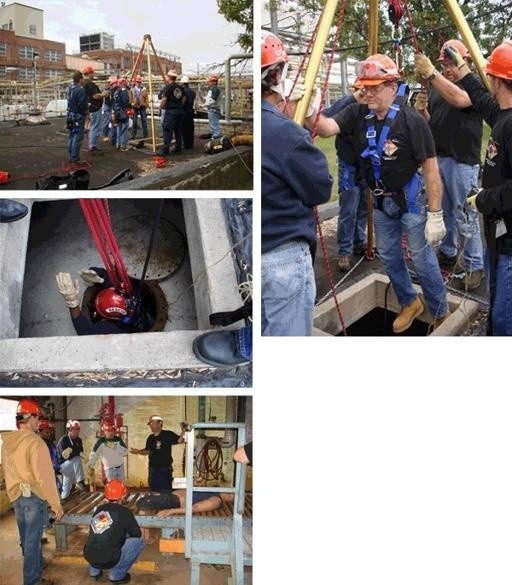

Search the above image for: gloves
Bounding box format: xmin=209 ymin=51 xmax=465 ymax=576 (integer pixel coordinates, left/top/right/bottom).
xmin=424 ymin=208 xmax=446 ymax=247
xmin=414 ymin=54 xmax=435 ymax=79
xmin=466 ymin=187 xmax=484 ymax=212
xmin=56 ymin=272 xmax=80 ymax=308
xmin=78 ymin=269 xmax=105 ymax=287
xmin=19 ymin=481 xmax=31 ymax=497
xmin=442 ymin=44 xmax=466 ymax=69
xmin=291 ymin=78 xmax=317 ymax=119
xmin=79 ymin=451 xmax=89 ymax=464
xmin=413 ymin=92 xmax=427 ymax=111
xmin=63 ymin=447 xmax=73 ymax=460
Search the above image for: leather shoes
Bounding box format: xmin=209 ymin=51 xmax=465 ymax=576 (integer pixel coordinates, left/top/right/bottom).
xmin=20 ymin=538 xmax=54 ymax=584
xmin=110 ymin=573 xmax=130 ymax=584
xmin=0 ymin=198 xmax=29 ymax=223
xmin=157 ymin=140 xmax=194 ymax=156
xmin=192 ymin=329 xmax=251 ymax=368
xmin=90 ymin=569 xmax=103 ymax=580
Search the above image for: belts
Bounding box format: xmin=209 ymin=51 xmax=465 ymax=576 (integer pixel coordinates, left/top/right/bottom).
xmin=208 ymin=106 xmax=217 ymax=109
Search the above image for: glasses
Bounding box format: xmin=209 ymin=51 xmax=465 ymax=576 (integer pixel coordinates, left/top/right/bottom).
xmin=440 ymin=63 xmax=455 ymax=68
xmin=354 ymin=61 xmax=399 ymax=79
xmin=362 ymin=83 xmax=390 ymax=93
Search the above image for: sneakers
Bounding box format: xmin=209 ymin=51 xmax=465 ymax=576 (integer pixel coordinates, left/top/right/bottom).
xmin=69 ymin=133 xmax=149 ymax=166
xmin=75 ymin=483 xmax=87 ymax=491
xmin=337 ymin=231 xmax=485 ymax=333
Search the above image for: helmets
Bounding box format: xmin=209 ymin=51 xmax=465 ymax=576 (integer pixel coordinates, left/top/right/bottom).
xmin=353 ymin=53 xmax=400 ymax=86
xmin=83 ymin=66 xmax=218 ymax=88
xmin=95 ymin=286 xmax=128 ymax=320
xmin=16 ymin=400 xmax=163 ymax=432
xmin=352 ymin=76 xmax=364 ymax=88
xmin=261 ymin=28 xmax=287 ymax=67
xmin=104 ymin=480 xmax=127 ymax=501
xmin=437 ymin=38 xmax=471 ymax=61
xmin=483 ymin=37 xmax=511 ymax=80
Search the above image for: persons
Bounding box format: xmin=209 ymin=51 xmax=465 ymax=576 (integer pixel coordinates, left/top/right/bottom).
xmin=137 ymin=490 xmax=235 ymax=518
xmin=56 ymin=267 xmax=155 ymax=335
xmin=1 ymin=399 xmax=65 ymax=585
xmin=56 ymin=420 xmax=85 ymax=499
xmin=129 ymin=414 xmax=188 ymax=491
xmin=198 ymin=74 xmax=221 ymax=139
xmin=83 ymin=478 xmax=146 ymax=583
xmin=192 ymin=323 xmax=252 ymax=369
xmin=66 ymin=65 xmax=195 ymax=168
xmin=89 ymin=418 xmax=128 ymax=485
xmin=37 ymin=420 xmax=63 ymax=543
xmin=261 ymin=30 xmax=334 ymax=336
xmin=233 ymin=441 xmax=252 ymax=468
xmin=284 ymin=38 xmax=512 ymax=336
